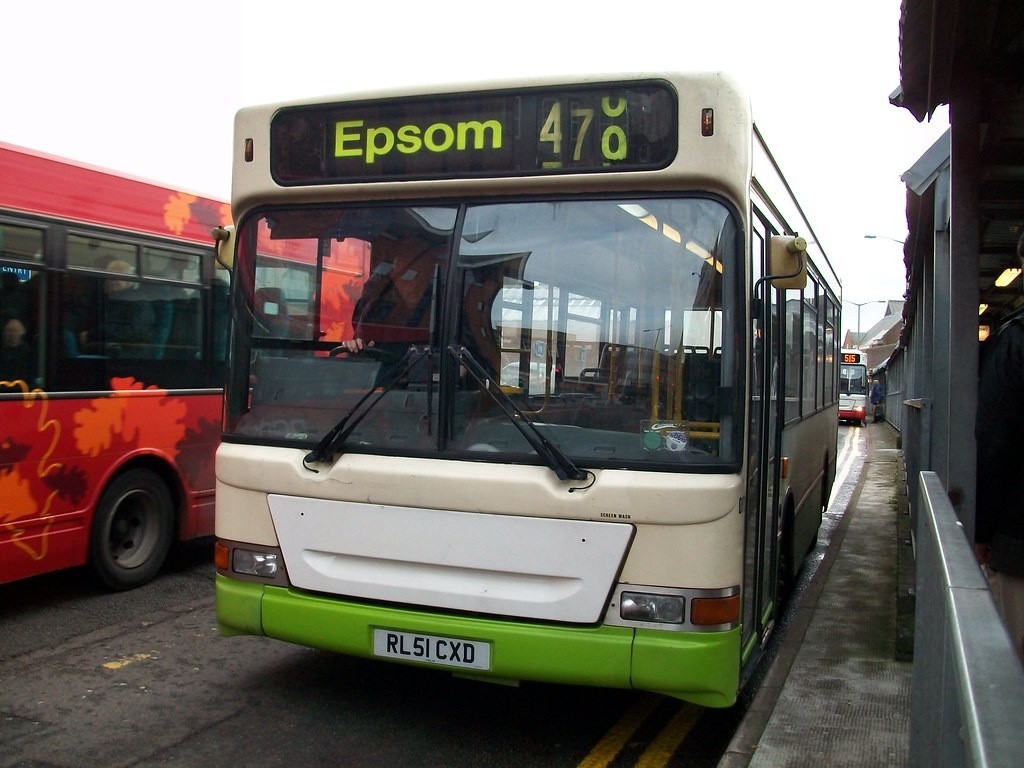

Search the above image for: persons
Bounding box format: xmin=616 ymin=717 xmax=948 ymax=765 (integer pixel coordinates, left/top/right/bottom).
xmin=870 ymin=379 xmax=886 ymax=423
xmin=0 ymin=258 xmax=160 ymax=398
xmin=974 ymin=229 xmax=1024 ymax=679
xmin=337 ymin=240 xmax=482 ymax=397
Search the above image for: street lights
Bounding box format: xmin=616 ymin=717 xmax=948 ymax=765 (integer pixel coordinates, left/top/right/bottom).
xmin=843 ymin=301 xmax=886 ymax=351
xmin=865 ymin=235 xmax=905 ymax=244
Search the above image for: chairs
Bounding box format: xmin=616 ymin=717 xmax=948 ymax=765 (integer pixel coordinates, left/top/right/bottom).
xmin=560 ymin=341 xmax=723 ymax=423
xmin=130 ymin=277 xmax=290 ymax=375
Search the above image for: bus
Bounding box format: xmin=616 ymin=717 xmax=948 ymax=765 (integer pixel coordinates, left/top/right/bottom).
xmin=838 ymin=349 xmax=873 ymax=426
xmin=215 ymin=71 xmax=843 ymax=708
xmin=0 ymin=142 xmax=503 ymax=596
